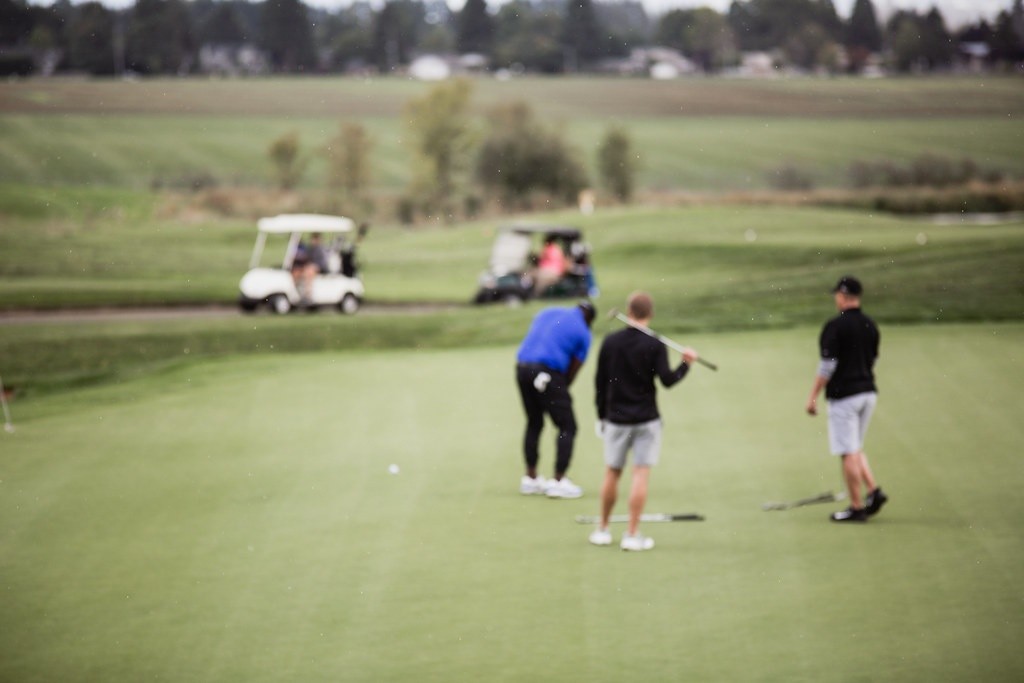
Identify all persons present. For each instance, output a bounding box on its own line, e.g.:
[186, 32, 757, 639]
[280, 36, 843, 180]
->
[590, 293, 694, 552]
[512, 300, 595, 497]
[807, 275, 889, 522]
[293, 232, 345, 290]
[529, 236, 568, 292]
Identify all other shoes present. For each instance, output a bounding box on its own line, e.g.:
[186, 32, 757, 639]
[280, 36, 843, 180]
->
[832, 506, 867, 520]
[621, 534, 655, 551]
[590, 531, 612, 545]
[864, 487, 887, 513]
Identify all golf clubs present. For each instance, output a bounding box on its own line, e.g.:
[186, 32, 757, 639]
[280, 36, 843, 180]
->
[577, 513, 704, 524]
[606, 307, 719, 374]
[763, 492, 835, 513]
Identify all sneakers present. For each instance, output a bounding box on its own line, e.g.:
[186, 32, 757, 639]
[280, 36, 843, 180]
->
[546, 477, 582, 498]
[521, 475, 547, 494]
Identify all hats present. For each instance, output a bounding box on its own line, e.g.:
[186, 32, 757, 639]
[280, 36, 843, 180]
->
[830, 275, 862, 295]
[579, 301, 595, 327]
